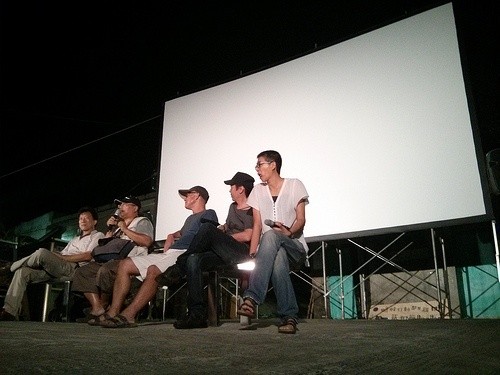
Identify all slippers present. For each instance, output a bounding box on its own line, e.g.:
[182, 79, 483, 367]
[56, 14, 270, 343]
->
[88, 311, 111, 325]
[102, 313, 137, 328]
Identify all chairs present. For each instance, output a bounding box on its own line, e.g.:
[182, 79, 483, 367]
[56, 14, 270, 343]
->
[43, 239, 87, 322]
[147, 239, 251, 329]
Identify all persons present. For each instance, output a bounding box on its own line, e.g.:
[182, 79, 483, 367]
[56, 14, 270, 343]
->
[155, 171, 255, 329]
[236, 150, 310, 334]
[71, 195, 153, 323]
[88, 186, 218, 328]
[0, 207, 105, 320]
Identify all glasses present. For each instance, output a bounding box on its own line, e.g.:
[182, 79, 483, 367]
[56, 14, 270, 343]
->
[255, 161, 273, 168]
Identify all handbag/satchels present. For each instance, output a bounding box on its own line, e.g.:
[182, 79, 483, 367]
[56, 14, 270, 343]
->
[90, 236, 134, 261]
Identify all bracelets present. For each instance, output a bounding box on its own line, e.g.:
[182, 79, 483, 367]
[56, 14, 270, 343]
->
[289, 231, 292, 236]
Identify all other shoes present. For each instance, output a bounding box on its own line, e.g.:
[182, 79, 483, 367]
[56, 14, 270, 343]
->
[75, 312, 95, 323]
[174, 313, 207, 329]
[0, 312, 12, 321]
[177, 251, 191, 263]
[0, 266, 9, 274]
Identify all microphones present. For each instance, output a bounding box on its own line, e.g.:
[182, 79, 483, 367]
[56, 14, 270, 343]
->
[264, 219, 290, 230]
[107, 209, 120, 230]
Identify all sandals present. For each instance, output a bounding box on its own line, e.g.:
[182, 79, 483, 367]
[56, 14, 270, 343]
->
[278, 319, 296, 334]
[238, 303, 255, 317]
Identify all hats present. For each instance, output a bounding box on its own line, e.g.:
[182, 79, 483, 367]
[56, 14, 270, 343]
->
[179, 185, 208, 202]
[224, 172, 254, 188]
[113, 196, 141, 209]
